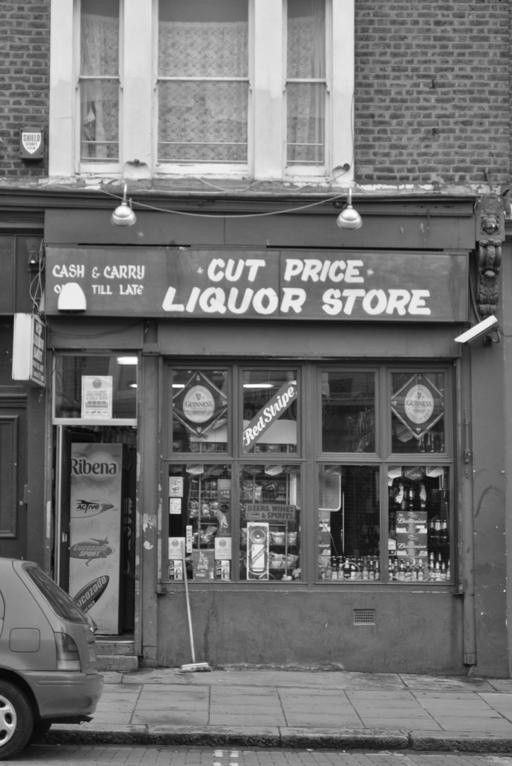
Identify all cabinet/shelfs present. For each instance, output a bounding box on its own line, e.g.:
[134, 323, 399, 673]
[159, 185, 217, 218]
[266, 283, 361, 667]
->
[186, 442, 299, 581]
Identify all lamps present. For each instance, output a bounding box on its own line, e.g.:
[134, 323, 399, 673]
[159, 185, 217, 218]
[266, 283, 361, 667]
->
[334, 188, 364, 232]
[111, 184, 137, 228]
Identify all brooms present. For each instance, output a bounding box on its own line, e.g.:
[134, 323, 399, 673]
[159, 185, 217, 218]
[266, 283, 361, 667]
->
[180, 541, 212, 672]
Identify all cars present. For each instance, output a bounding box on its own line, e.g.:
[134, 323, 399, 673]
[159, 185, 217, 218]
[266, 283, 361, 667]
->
[0, 553, 103, 760]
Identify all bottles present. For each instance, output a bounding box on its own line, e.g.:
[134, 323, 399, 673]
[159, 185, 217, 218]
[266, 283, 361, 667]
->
[397, 483, 428, 511]
[329, 551, 451, 582]
[428, 518, 449, 540]
[418, 431, 445, 452]
